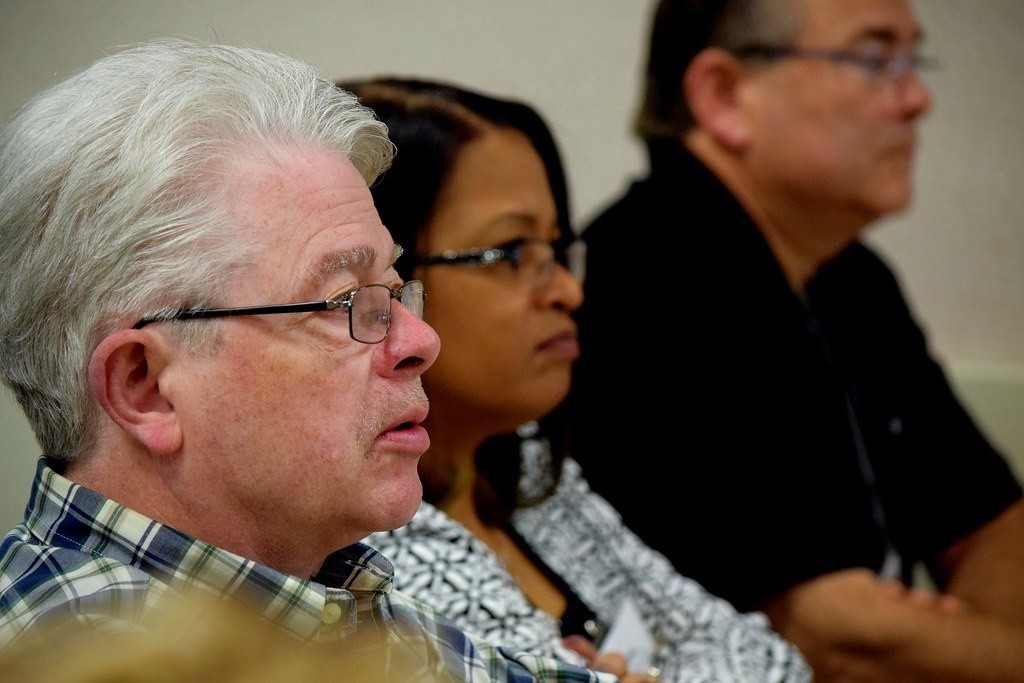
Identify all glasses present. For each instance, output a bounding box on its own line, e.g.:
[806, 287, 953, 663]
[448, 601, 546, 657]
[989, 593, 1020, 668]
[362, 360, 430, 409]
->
[132, 279, 426, 344]
[736, 43, 941, 97]
[405, 236, 589, 284]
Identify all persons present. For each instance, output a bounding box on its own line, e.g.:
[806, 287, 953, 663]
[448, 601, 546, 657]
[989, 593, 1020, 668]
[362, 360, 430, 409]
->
[534, 2, 1022, 683]
[333, 76, 815, 683]
[0, 39, 600, 683]
